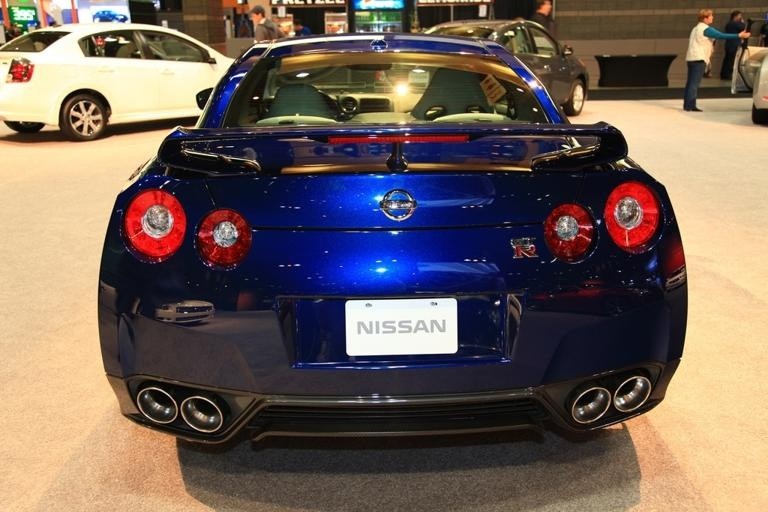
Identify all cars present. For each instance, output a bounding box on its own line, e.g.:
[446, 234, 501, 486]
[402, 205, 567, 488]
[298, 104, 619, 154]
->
[407, 17, 590, 117]
[0, 21, 237, 143]
[730, 16, 767, 127]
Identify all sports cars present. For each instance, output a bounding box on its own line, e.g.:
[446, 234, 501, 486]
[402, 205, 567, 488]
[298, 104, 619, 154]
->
[95, 33, 689, 447]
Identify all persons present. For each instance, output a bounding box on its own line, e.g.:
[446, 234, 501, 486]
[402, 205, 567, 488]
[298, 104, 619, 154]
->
[720, 10, 745, 79]
[294, 19, 312, 36]
[249, 5, 286, 41]
[760, 23, 768, 47]
[682, 8, 751, 112]
[528, 0, 552, 29]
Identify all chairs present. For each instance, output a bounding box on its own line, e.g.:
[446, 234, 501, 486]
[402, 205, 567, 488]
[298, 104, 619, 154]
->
[256, 67, 519, 124]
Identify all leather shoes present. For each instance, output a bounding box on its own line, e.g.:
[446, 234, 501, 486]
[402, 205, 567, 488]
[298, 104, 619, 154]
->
[684, 106, 703, 112]
